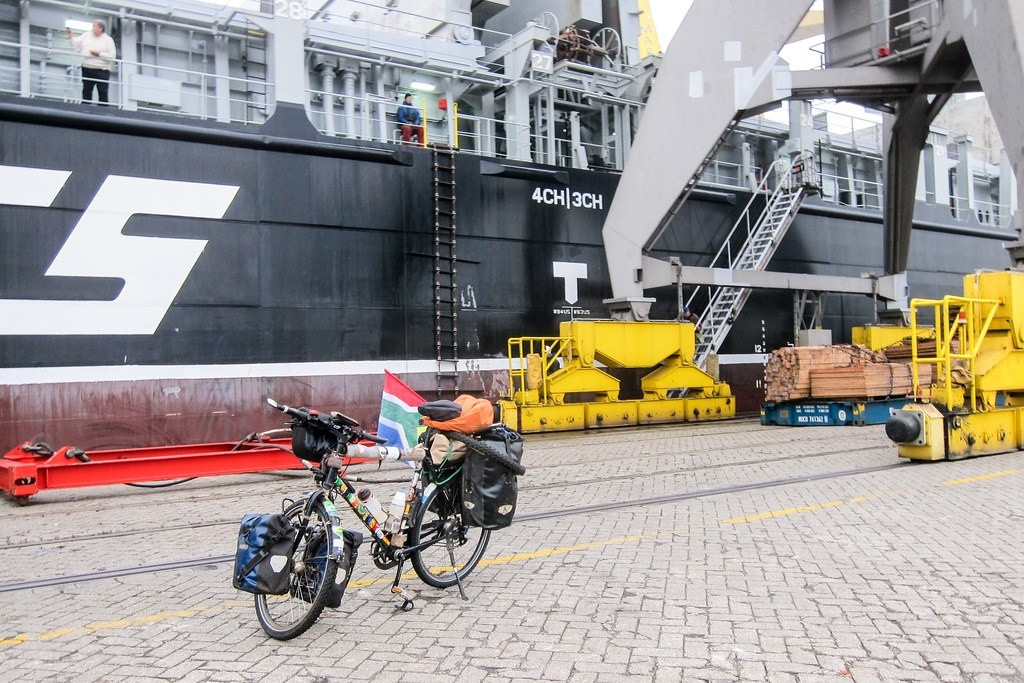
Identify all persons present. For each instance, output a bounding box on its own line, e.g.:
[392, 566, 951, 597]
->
[397, 90, 425, 145]
[63, 20, 117, 106]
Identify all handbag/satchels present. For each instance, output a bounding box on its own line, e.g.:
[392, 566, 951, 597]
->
[292, 529, 363, 608]
[460, 436, 525, 530]
[230, 511, 291, 595]
[290, 407, 347, 463]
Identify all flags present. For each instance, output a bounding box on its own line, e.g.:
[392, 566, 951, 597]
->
[373, 370, 433, 470]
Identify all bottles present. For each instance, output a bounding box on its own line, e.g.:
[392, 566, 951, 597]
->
[358, 488, 388, 524]
[385, 491, 406, 533]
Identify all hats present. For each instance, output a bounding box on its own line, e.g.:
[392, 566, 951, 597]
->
[405, 93, 412, 100]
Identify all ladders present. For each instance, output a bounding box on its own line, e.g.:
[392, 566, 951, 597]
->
[431, 145, 460, 399]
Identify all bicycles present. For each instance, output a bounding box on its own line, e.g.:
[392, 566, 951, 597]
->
[254, 400, 525, 643]
